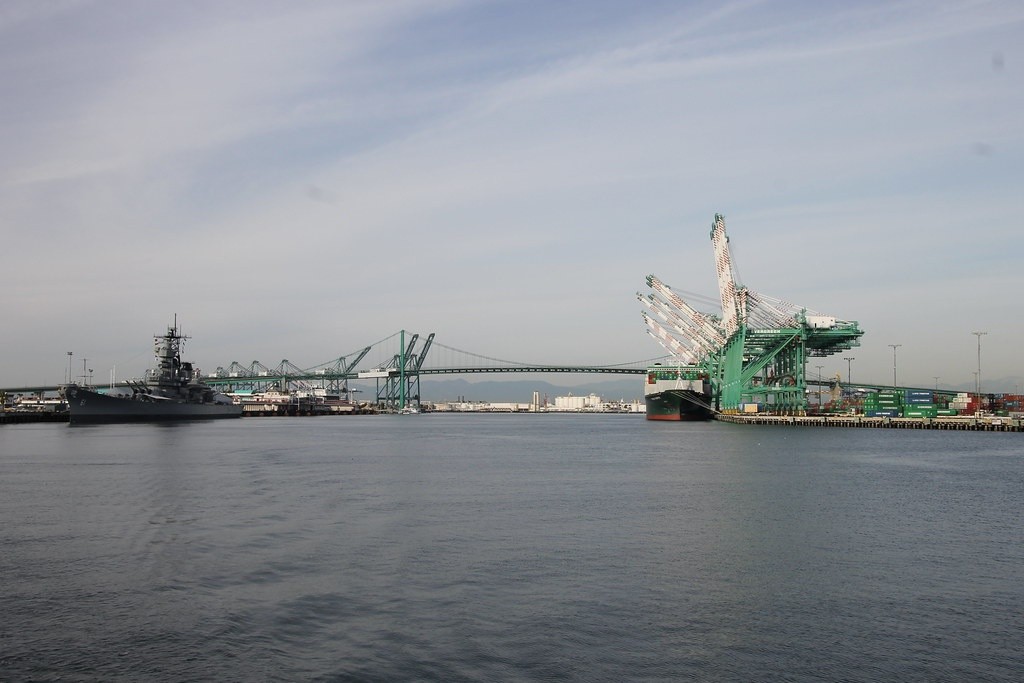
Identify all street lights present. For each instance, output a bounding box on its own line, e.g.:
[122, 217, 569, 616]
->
[972, 332, 987, 412]
[815, 365, 824, 405]
[933, 377, 940, 395]
[844, 357, 855, 404]
[888, 344, 902, 393]
[972, 371, 978, 397]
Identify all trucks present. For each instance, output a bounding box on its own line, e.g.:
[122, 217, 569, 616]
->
[805, 316, 839, 330]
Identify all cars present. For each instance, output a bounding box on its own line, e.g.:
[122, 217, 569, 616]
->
[835, 319, 854, 330]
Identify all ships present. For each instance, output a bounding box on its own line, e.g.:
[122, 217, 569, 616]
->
[58, 313, 245, 426]
[645, 361, 712, 421]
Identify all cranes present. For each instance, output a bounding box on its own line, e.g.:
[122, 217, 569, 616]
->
[636, 212, 866, 418]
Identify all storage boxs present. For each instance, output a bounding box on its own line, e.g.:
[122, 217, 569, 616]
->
[863, 390, 1024, 418]
[806, 399, 863, 415]
[648, 371, 710, 384]
